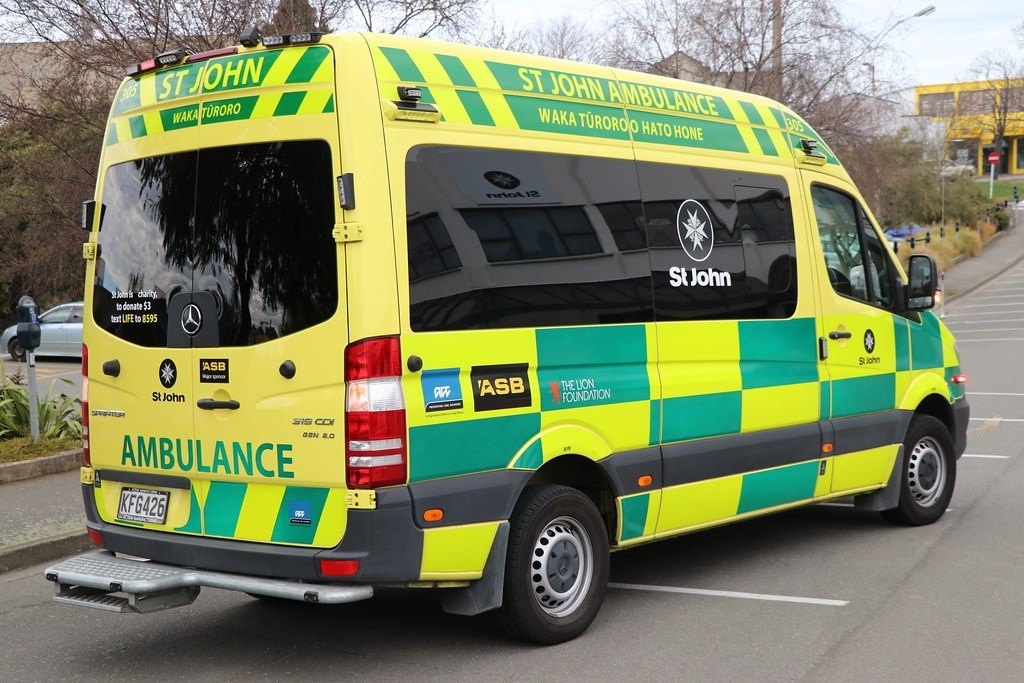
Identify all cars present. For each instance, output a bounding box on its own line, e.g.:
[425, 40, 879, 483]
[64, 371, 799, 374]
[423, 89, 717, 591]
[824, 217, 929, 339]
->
[0, 302, 84, 363]
[883, 221, 924, 237]
[820, 238, 871, 267]
[924, 159, 975, 181]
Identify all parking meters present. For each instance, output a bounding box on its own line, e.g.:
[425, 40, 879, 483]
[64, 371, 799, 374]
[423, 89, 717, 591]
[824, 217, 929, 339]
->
[15, 296, 41, 439]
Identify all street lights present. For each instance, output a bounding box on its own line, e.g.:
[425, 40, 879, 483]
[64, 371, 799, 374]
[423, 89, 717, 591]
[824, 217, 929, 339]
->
[871, 6, 935, 223]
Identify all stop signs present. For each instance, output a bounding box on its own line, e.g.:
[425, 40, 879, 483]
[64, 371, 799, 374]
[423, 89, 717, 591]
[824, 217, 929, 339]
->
[988, 152, 999, 164]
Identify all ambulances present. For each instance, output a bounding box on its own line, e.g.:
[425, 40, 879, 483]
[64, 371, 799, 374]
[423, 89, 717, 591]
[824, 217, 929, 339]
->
[44, 27, 970, 645]
